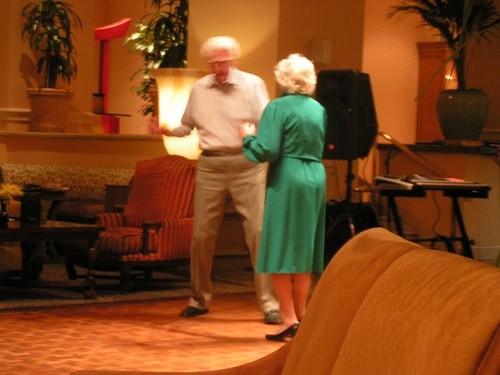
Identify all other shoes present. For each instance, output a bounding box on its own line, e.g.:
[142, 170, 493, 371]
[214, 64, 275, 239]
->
[180, 306, 208, 318]
[264, 311, 284, 324]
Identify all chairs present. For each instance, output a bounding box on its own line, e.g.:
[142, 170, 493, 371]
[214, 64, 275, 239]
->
[214, 228, 500, 375]
[87, 156, 198, 285]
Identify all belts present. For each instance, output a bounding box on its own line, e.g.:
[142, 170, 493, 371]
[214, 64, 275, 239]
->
[201, 150, 242, 157]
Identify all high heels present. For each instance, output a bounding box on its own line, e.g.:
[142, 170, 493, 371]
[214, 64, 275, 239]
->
[266, 323, 299, 340]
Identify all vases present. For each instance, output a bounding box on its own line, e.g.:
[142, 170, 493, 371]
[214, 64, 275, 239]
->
[0, 198, 9, 222]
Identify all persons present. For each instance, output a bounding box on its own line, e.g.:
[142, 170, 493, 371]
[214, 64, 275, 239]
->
[151, 36, 287, 324]
[239, 54, 328, 341]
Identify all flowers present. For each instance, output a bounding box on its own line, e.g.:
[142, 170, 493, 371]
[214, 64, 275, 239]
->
[0, 182, 25, 201]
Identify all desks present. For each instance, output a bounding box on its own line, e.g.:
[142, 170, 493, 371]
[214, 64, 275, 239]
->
[351, 182, 491, 258]
[0, 220, 105, 298]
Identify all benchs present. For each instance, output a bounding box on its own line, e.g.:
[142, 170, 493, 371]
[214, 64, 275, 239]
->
[0, 162, 135, 253]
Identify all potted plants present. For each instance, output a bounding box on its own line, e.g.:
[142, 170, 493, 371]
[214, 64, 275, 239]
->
[25, 0, 82, 131]
[385, 0, 500, 141]
